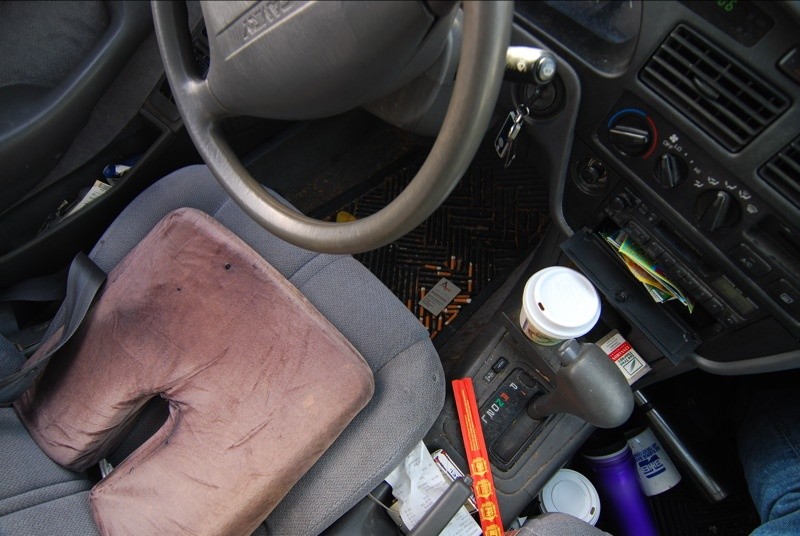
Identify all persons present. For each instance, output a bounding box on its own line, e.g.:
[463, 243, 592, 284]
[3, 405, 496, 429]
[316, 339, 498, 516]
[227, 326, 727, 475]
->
[734, 388, 800, 536]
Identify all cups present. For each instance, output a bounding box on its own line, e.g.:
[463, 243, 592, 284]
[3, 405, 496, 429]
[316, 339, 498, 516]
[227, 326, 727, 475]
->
[628, 427, 682, 496]
[537, 469, 601, 526]
[583, 442, 659, 536]
[520, 265, 601, 346]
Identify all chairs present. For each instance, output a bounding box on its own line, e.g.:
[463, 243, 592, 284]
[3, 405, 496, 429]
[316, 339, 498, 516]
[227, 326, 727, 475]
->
[0, 164, 446, 536]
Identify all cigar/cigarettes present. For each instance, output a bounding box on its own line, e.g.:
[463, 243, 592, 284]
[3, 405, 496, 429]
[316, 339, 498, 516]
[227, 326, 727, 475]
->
[407, 255, 472, 339]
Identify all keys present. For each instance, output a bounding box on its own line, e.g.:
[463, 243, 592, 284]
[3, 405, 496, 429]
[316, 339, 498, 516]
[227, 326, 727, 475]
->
[521, 85, 550, 112]
